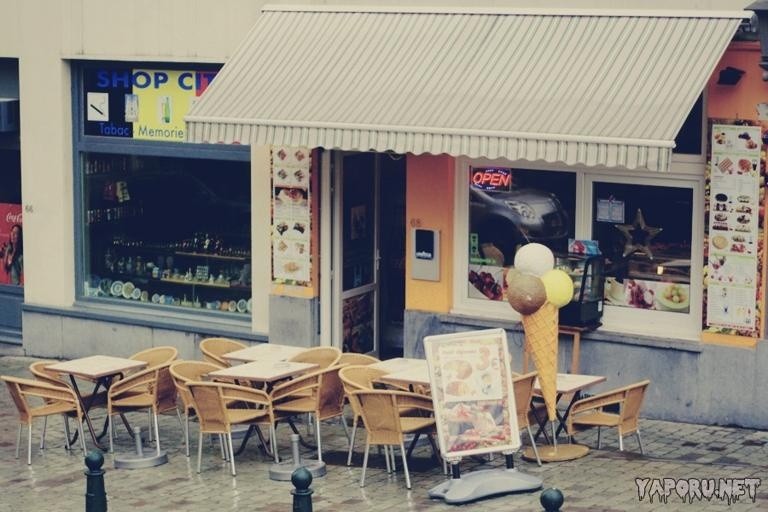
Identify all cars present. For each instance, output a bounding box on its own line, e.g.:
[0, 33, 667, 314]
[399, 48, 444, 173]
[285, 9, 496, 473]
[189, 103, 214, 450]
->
[470, 184, 571, 240]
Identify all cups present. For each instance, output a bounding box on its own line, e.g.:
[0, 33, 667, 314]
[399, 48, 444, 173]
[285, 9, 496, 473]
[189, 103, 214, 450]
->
[611, 278, 629, 302]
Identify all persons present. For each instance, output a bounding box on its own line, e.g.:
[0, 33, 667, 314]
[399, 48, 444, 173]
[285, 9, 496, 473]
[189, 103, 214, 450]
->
[0, 223, 23, 285]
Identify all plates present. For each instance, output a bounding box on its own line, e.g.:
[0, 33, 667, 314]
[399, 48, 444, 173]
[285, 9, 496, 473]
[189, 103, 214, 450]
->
[656, 284, 689, 309]
[606, 285, 635, 307]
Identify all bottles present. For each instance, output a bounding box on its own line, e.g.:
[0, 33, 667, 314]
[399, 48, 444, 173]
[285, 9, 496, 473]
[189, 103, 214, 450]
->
[85, 157, 145, 224]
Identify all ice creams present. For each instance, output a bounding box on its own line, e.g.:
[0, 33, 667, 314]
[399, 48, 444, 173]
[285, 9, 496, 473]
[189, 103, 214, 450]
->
[507, 243, 574, 423]
